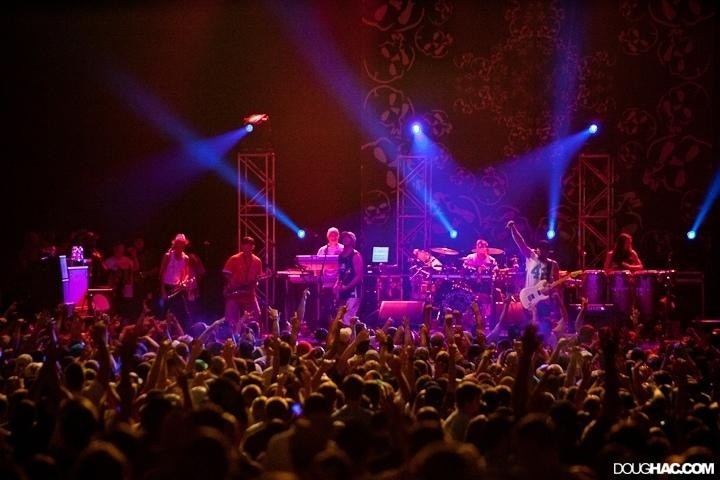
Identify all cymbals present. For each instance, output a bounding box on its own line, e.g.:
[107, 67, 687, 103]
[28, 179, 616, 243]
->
[431, 247, 458, 256]
[472, 248, 504, 255]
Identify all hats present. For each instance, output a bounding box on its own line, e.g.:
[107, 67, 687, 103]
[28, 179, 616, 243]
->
[327, 227, 339, 239]
[171, 233, 189, 246]
[340, 231, 356, 242]
[534, 239, 554, 254]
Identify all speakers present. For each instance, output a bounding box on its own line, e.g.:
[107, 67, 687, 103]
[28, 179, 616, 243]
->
[568, 302, 630, 335]
[379, 300, 426, 328]
[38, 255, 91, 318]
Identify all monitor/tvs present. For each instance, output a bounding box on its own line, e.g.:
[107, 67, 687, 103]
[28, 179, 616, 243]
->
[372, 246, 390, 264]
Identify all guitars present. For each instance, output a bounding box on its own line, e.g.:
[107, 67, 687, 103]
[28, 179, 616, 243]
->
[519, 270, 583, 310]
[154, 278, 196, 311]
[224, 268, 272, 295]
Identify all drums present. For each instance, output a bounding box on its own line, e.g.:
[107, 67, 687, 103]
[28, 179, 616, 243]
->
[442, 284, 475, 314]
[582, 269, 608, 304]
[606, 271, 634, 314]
[656, 270, 675, 299]
[632, 269, 658, 316]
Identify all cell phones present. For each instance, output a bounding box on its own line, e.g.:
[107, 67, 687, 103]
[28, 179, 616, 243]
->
[291, 402, 303, 416]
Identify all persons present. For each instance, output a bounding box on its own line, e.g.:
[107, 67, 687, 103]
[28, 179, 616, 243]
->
[1, 216, 720, 480]
